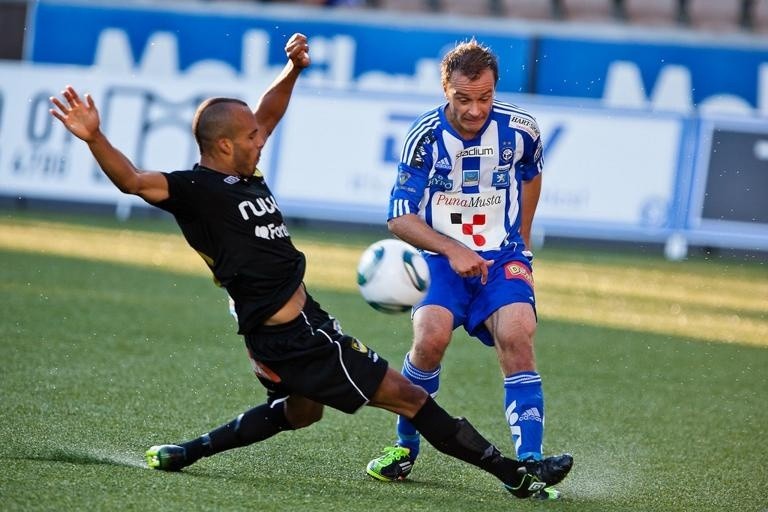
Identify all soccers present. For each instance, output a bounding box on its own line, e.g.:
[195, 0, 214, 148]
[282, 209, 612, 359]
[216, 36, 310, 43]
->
[356, 239, 430, 313]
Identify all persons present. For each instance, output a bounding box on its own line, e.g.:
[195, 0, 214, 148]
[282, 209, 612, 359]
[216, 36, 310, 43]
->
[46, 28, 576, 503]
[365, 36, 571, 502]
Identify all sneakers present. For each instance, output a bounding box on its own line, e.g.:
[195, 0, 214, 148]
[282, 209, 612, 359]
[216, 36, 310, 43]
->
[503, 453, 574, 501]
[145, 444, 185, 470]
[366, 446, 414, 482]
